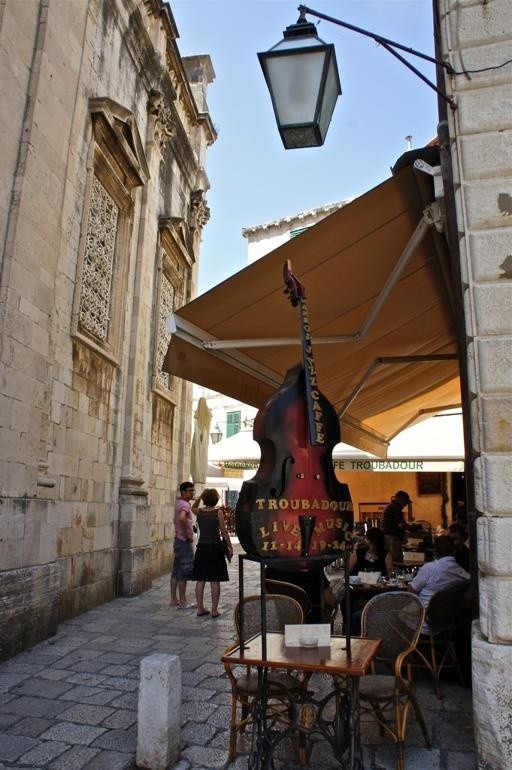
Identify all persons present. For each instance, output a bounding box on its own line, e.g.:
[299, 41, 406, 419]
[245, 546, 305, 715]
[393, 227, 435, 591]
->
[168, 482, 197, 609]
[448, 524, 469, 574]
[191, 488, 233, 617]
[388, 535, 472, 675]
[349, 528, 393, 636]
[383, 490, 413, 561]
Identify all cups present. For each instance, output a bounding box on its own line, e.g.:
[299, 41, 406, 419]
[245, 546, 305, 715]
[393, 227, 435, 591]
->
[349, 568, 413, 588]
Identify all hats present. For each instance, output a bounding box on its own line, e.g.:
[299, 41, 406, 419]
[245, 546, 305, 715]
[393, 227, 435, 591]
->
[395, 490, 412, 504]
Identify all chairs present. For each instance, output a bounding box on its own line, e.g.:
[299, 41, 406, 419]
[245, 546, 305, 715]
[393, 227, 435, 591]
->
[416, 520, 431, 531]
[265, 579, 313, 624]
[221, 594, 312, 769]
[406, 579, 467, 699]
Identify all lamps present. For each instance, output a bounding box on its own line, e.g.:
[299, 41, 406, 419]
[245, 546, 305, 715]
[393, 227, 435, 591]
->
[258, 2, 457, 151]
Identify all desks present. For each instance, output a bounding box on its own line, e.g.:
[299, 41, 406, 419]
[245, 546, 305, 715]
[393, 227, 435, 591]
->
[221, 630, 383, 770]
[335, 591, 432, 770]
[343, 579, 411, 593]
[392, 552, 425, 572]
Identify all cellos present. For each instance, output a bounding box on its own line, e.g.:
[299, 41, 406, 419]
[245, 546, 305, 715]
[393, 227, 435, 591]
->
[235, 260, 354, 573]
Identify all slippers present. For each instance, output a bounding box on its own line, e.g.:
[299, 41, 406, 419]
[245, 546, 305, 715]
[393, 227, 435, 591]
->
[212, 610, 221, 618]
[168, 601, 180, 606]
[177, 602, 197, 609]
[197, 609, 210, 616]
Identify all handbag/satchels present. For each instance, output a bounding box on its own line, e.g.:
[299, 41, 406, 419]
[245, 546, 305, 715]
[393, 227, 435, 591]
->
[221, 537, 232, 563]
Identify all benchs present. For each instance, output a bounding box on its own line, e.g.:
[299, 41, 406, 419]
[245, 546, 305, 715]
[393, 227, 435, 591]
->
[402, 536, 424, 551]
